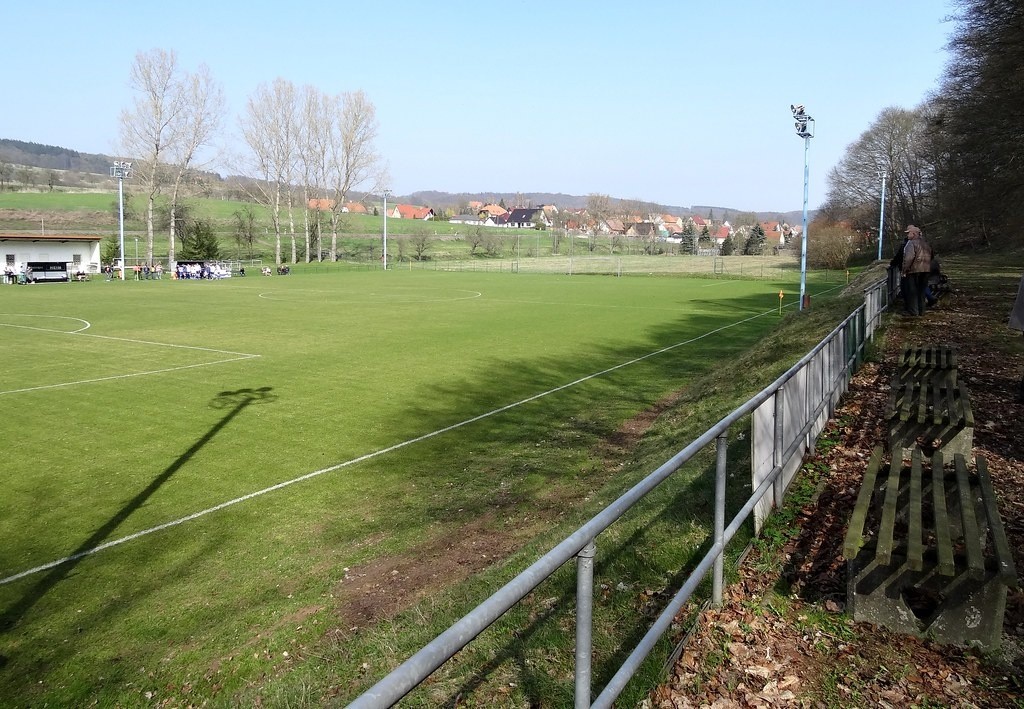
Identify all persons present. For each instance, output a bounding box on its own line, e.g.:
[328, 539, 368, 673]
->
[176, 263, 221, 280]
[277, 265, 290, 275]
[264, 267, 272, 276]
[4, 263, 33, 285]
[77, 271, 85, 281]
[890, 225, 941, 317]
[133, 261, 163, 280]
[105, 264, 113, 281]
[240, 268, 245, 277]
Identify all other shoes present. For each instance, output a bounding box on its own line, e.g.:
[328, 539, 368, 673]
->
[902, 311, 919, 317]
[925, 298, 938, 306]
[918, 309, 925, 316]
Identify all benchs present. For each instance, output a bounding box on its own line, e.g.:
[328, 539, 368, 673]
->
[886, 373, 975, 467]
[899, 345, 958, 389]
[843, 446, 1016, 651]
[73, 273, 90, 282]
[930, 275, 951, 305]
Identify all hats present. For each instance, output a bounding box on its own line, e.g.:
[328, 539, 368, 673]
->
[904, 227, 921, 233]
